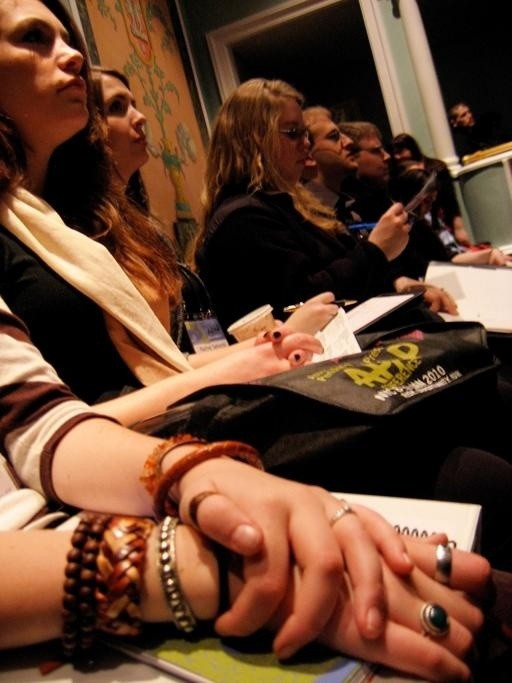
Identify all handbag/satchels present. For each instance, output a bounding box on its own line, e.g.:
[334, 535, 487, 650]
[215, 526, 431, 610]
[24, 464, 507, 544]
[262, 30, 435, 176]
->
[159, 321, 501, 481]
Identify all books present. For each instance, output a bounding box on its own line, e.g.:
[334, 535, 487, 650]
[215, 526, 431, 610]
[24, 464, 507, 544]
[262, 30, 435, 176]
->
[116, 626, 368, 683]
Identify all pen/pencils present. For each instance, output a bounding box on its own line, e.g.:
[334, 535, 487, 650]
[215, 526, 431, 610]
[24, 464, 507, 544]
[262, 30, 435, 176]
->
[283, 299, 358, 313]
[348, 218, 415, 231]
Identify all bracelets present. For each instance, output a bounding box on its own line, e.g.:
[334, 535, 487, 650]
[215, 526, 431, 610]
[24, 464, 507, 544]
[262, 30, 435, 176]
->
[59, 432, 264, 668]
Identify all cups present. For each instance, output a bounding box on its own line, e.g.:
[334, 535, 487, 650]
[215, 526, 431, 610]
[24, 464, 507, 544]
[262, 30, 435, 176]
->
[227, 303, 277, 344]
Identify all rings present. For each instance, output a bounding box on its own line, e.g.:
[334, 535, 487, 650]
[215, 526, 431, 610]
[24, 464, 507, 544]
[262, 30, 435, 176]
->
[434, 540, 452, 583]
[328, 504, 354, 525]
[419, 600, 451, 640]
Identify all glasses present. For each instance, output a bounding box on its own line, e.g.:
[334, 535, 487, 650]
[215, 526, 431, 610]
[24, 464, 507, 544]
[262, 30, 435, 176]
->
[278, 126, 299, 139]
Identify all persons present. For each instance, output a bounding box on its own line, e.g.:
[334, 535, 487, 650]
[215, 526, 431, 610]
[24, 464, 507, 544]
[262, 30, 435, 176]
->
[0, 303, 493, 683]
[300, 101, 512, 279]
[196, 79, 459, 346]
[0, 4, 328, 426]
[52, 63, 339, 371]
